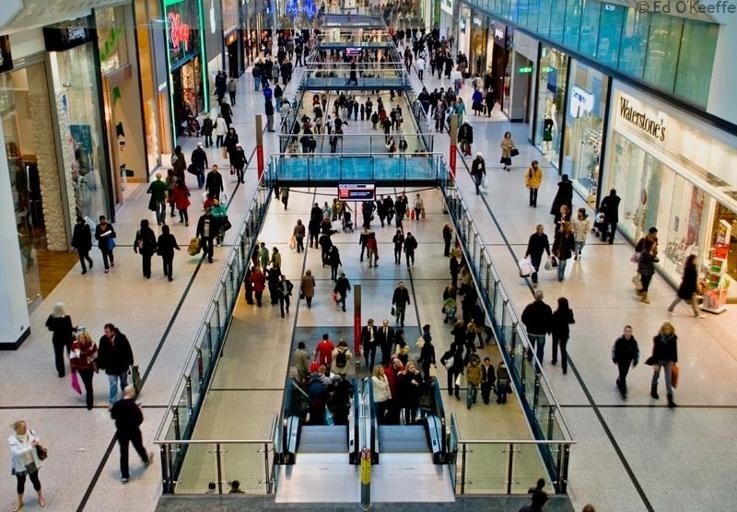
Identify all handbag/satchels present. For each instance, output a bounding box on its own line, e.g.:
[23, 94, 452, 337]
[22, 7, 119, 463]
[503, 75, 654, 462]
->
[188, 238, 201, 256]
[36, 444, 48, 460]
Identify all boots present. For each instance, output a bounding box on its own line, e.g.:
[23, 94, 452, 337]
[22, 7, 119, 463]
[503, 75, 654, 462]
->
[667, 393, 678, 409]
[651, 384, 659, 399]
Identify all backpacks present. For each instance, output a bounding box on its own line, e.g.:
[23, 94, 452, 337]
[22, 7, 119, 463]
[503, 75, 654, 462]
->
[336, 348, 347, 368]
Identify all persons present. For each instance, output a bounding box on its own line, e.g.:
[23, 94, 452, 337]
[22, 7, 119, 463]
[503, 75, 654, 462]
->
[645, 322, 678, 408]
[6, 420, 45, 512]
[667, 254, 708, 318]
[44, 0, 660, 511]
